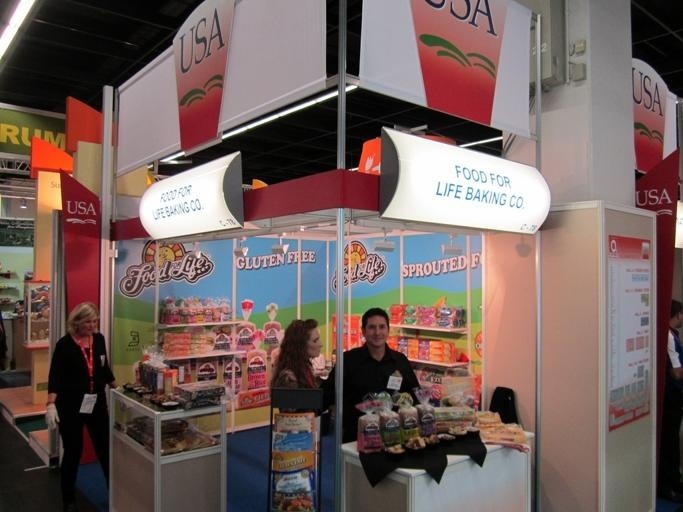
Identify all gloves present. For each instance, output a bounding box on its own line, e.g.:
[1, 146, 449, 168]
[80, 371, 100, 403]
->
[45, 404, 60, 430]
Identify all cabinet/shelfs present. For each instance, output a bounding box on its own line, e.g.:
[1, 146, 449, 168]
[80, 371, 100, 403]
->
[389, 322, 469, 368]
[157, 320, 247, 362]
[0, 272, 16, 305]
[22, 280, 52, 349]
[109, 388, 227, 512]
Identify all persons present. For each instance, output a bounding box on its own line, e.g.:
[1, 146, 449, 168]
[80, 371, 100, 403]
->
[45, 302, 118, 512]
[271, 319, 330, 418]
[15, 300, 25, 313]
[295, 308, 421, 443]
[658, 299, 683, 504]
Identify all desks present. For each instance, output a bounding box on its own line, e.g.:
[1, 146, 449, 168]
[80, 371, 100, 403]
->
[339, 431, 535, 512]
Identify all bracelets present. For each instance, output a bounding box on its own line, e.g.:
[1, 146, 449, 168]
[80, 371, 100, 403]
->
[47, 401, 56, 406]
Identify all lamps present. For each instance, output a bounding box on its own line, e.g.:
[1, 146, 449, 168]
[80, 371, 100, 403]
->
[189, 232, 463, 259]
